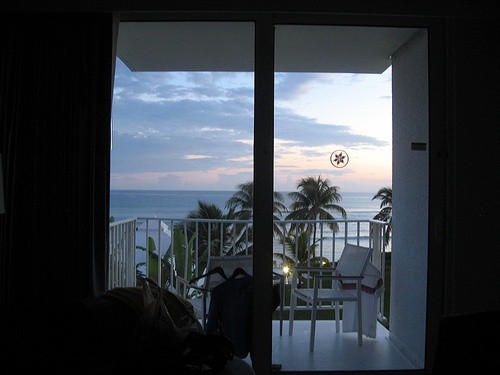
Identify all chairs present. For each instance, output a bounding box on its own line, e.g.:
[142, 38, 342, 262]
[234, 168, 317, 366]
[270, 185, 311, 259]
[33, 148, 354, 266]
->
[287, 244, 375, 352]
[173, 253, 257, 328]
